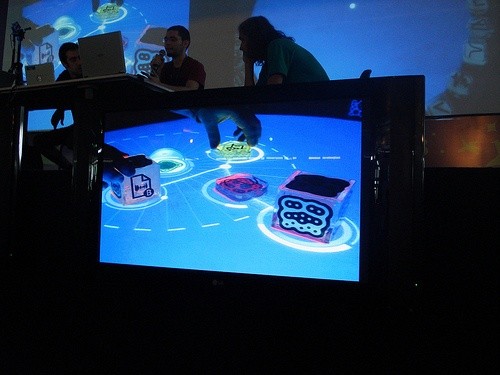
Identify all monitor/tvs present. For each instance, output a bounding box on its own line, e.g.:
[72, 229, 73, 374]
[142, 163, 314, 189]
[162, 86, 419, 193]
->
[25, 63, 56, 85]
[92, 74, 424, 295]
[78, 31, 126, 78]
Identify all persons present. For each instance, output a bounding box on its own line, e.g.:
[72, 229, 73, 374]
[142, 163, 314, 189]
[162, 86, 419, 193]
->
[239, 16, 329, 86]
[51, 42, 100, 134]
[142, 25, 206, 91]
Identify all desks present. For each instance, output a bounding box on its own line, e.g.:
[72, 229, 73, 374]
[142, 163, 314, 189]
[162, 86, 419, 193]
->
[0, 73, 175, 242]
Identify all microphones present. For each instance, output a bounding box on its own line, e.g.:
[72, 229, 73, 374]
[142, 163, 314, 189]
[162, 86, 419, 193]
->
[154, 50, 165, 70]
